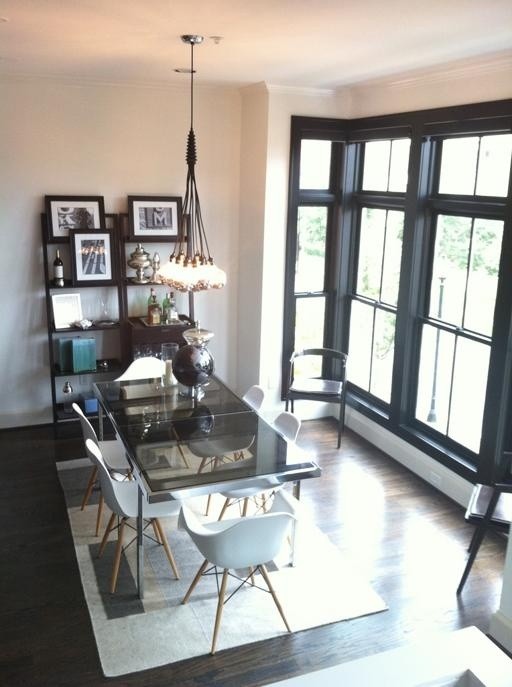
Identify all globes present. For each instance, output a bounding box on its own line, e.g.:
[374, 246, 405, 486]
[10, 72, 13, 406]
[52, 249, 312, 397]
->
[171, 399, 215, 442]
[172, 344, 213, 398]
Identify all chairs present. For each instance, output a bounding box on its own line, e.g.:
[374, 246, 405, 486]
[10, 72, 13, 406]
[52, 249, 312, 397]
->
[188, 384, 262, 478]
[72, 401, 159, 536]
[287, 347, 350, 447]
[176, 489, 299, 656]
[112, 355, 188, 469]
[211, 410, 300, 520]
[75, 439, 182, 596]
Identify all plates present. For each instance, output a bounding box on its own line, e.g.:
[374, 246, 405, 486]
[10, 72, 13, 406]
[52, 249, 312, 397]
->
[93, 319, 115, 328]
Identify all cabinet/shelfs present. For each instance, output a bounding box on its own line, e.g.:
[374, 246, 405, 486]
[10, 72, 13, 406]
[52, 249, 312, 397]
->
[42, 215, 129, 437]
[114, 213, 194, 365]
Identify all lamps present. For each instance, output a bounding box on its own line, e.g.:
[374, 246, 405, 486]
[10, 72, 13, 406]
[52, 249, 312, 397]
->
[156, 34, 227, 293]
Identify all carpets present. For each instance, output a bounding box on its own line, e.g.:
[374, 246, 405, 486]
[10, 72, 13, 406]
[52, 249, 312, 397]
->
[53, 453, 388, 679]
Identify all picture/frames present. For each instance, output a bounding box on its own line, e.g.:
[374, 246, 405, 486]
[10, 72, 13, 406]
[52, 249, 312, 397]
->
[44, 195, 105, 243]
[67, 227, 118, 283]
[127, 194, 183, 239]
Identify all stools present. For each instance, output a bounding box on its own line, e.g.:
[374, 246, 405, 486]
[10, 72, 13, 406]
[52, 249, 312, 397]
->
[452, 451, 511, 594]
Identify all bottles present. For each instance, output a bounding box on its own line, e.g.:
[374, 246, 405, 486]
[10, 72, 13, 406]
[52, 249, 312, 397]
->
[147, 288, 179, 325]
[53, 249, 65, 287]
[64, 381, 74, 414]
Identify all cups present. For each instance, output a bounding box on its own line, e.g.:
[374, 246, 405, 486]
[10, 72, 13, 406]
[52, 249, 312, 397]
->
[134, 344, 160, 359]
[160, 387, 179, 425]
[161, 342, 179, 387]
